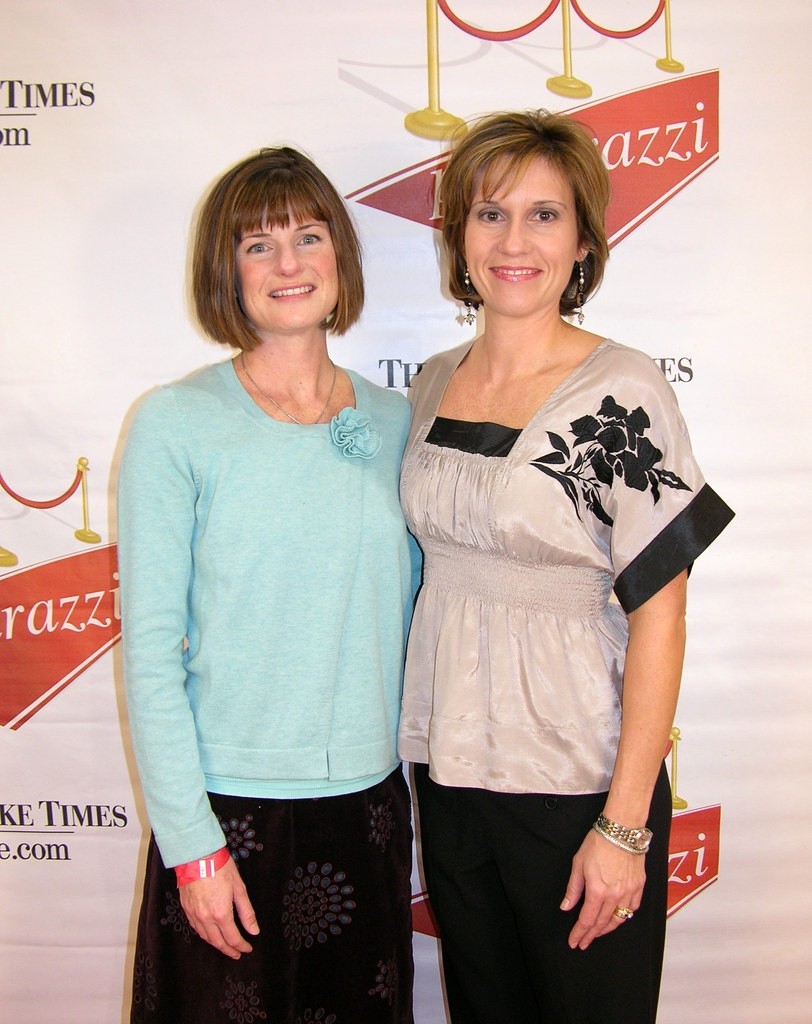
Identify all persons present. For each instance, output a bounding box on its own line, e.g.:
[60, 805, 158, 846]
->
[400, 109, 734, 1023]
[117, 147, 424, 1024]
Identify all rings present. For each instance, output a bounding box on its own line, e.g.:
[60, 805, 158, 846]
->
[615, 908, 633, 919]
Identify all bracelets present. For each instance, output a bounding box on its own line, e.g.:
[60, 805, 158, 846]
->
[173, 847, 231, 888]
[593, 823, 649, 854]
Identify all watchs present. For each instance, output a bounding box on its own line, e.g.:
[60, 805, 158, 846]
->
[597, 812, 653, 850]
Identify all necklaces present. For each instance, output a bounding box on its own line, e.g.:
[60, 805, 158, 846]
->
[241, 350, 336, 425]
